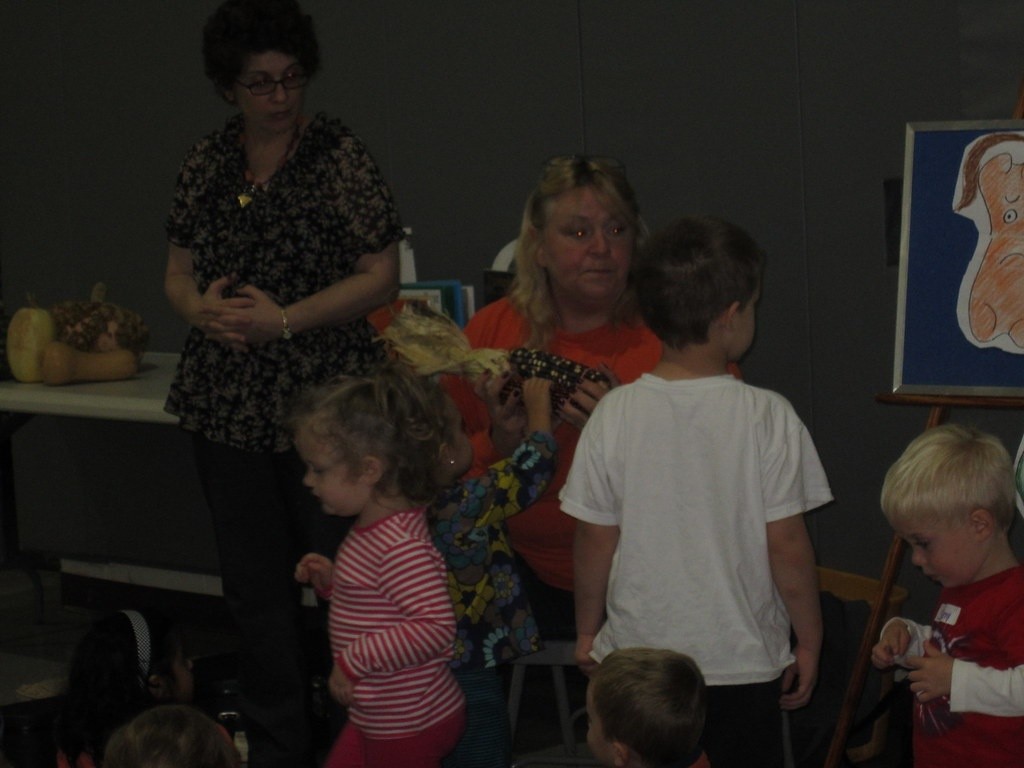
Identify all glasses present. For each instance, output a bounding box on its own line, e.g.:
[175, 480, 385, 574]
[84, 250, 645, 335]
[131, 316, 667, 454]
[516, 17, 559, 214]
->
[231, 62, 312, 96]
[540, 154, 627, 189]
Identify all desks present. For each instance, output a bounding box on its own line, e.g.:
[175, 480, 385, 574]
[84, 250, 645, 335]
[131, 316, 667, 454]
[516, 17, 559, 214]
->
[0, 351, 179, 566]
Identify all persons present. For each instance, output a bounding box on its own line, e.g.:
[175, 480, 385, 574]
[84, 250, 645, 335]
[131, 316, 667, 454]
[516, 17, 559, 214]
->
[587, 648, 710, 768]
[438, 156, 742, 768]
[559, 219, 834, 768]
[293, 361, 467, 768]
[416, 379, 558, 768]
[872, 425, 1024, 768]
[104, 707, 240, 768]
[164, 0, 404, 768]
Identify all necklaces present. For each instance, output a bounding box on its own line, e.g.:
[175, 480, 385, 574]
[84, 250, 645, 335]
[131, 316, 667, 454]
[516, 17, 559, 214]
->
[240, 124, 300, 186]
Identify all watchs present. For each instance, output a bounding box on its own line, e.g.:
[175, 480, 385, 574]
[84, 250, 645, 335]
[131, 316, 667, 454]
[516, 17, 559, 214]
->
[280, 308, 292, 342]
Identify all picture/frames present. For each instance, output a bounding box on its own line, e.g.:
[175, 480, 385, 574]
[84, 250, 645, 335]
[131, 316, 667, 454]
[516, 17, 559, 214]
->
[890, 118, 1024, 397]
